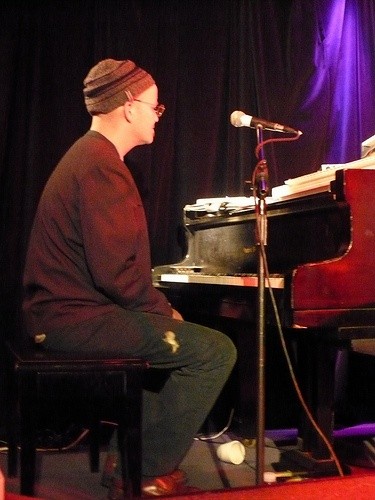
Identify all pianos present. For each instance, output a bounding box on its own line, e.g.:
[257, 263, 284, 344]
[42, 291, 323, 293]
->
[152, 168, 375, 463]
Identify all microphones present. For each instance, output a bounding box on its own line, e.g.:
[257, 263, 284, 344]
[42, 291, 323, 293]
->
[230, 110, 298, 135]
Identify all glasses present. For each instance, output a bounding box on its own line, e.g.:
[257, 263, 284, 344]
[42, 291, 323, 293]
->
[133, 98, 165, 117]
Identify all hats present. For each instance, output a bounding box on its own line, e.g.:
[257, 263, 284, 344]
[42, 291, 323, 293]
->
[83, 59, 155, 113]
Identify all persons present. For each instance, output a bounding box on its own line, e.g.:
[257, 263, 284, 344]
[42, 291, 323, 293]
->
[22, 57, 238, 497]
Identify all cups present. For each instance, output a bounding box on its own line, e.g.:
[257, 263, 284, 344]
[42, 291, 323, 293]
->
[216, 440, 245, 465]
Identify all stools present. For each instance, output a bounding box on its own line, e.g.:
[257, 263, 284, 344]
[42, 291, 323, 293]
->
[6, 341, 151, 500]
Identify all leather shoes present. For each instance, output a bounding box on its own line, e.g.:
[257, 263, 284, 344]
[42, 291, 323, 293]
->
[107, 477, 202, 499]
[100, 454, 188, 488]
